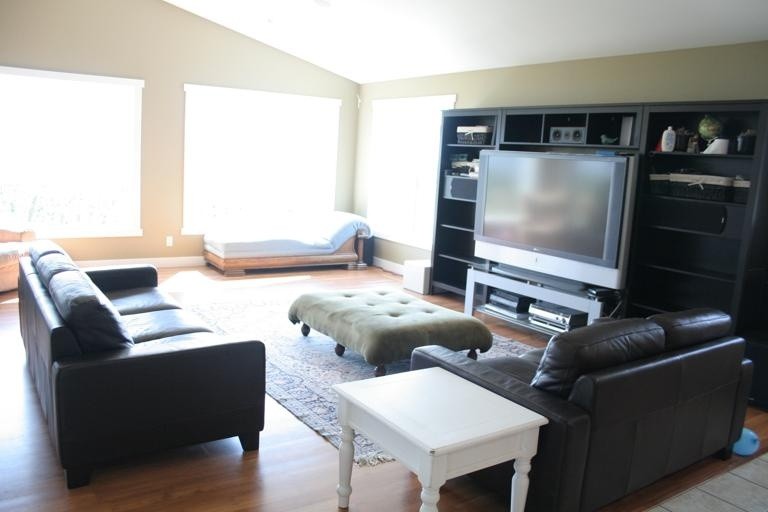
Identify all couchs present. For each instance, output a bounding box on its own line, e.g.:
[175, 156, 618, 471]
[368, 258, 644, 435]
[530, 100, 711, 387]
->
[0, 229, 33, 292]
[285, 284, 493, 378]
[410, 309, 754, 509]
[10, 242, 266, 491]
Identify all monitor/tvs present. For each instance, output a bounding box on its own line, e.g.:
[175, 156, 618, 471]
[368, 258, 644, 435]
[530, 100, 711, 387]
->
[473, 149, 638, 293]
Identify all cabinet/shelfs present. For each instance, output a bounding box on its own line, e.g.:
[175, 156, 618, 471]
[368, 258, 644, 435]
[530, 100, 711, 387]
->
[499, 103, 643, 154]
[462, 268, 626, 339]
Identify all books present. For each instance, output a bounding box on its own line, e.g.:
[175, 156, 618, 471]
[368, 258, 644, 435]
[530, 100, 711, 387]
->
[482, 289, 529, 320]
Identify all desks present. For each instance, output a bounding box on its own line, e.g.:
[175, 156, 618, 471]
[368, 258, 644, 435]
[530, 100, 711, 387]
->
[330, 368, 549, 510]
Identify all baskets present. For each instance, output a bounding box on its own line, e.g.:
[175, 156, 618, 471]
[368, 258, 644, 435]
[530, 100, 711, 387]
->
[456, 126, 493, 145]
[732, 179, 751, 204]
[668, 173, 734, 202]
[649, 174, 670, 195]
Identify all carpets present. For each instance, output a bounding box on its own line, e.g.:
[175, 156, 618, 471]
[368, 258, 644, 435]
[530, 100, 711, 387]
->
[188, 300, 537, 468]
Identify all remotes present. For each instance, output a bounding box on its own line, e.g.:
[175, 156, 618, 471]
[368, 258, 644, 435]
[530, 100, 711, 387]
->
[586, 287, 615, 295]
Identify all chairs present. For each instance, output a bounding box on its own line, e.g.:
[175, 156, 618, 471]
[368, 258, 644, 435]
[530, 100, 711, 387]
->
[623, 98, 766, 411]
[428, 106, 500, 300]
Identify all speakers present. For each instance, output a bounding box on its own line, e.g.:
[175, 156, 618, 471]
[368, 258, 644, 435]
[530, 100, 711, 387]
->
[549, 126, 586, 143]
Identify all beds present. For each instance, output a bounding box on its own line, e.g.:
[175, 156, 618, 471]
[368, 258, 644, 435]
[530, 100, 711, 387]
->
[201, 213, 372, 278]
